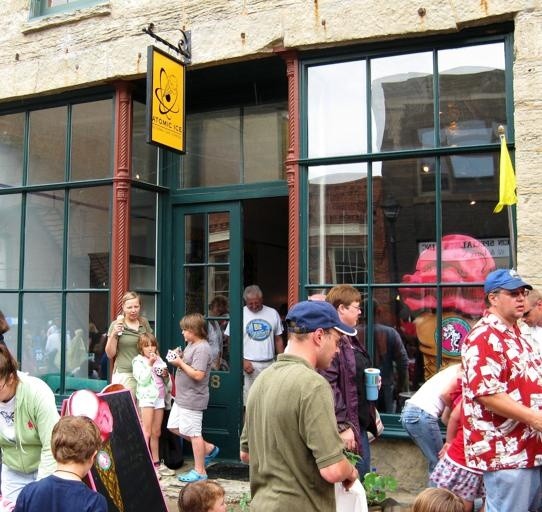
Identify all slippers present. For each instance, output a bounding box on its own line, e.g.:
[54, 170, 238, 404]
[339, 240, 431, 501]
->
[178, 469, 210, 483]
[203, 444, 220, 467]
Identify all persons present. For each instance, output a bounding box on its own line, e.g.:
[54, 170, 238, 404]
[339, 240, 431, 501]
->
[351, 294, 416, 423]
[166, 312, 219, 482]
[238, 303, 358, 511]
[460, 268, 541, 512]
[401, 363, 466, 491]
[177, 480, 227, 512]
[45, 318, 70, 375]
[1, 342, 61, 512]
[519, 285, 540, 356]
[313, 288, 381, 488]
[12, 416, 108, 512]
[132, 332, 177, 480]
[206, 296, 227, 371]
[412, 487, 465, 512]
[225, 285, 285, 424]
[428, 396, 488, 512]
[105, 292, 152, 426]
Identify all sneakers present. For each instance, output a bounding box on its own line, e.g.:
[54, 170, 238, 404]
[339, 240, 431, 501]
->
[155, 461, 176, 476]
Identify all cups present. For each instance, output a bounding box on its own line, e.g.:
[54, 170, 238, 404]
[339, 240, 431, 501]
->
[156, 366, 165, 375]
[363, 366, 381, 401]
[165, 349, 177, 361]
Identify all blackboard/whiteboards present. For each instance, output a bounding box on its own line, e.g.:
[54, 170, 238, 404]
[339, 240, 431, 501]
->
[66, 388, 169, 512]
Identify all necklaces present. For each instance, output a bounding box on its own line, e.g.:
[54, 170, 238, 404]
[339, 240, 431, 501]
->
[53, 470, 82, 480]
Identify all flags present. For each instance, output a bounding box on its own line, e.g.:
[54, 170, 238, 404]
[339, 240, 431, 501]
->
[493, 132, 519, 215]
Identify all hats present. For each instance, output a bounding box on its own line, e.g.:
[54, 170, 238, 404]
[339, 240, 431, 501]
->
[283, 297, 362, 338]
[482, 268, 536, 293]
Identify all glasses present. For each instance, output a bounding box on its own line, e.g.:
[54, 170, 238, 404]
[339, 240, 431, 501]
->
[494, 290, 531, 298]
